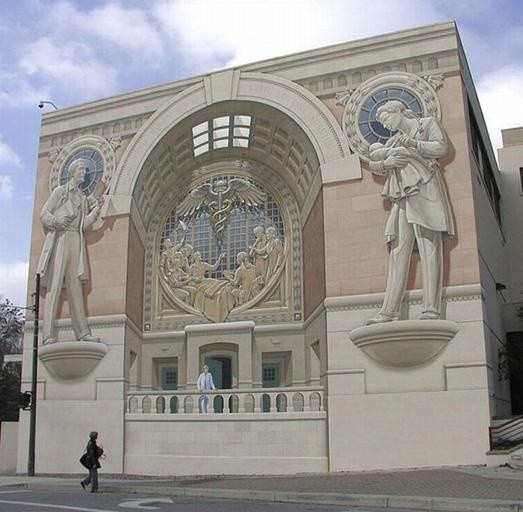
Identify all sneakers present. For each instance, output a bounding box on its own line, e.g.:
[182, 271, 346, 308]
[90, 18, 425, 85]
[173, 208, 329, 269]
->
[80, 481, 85, 489]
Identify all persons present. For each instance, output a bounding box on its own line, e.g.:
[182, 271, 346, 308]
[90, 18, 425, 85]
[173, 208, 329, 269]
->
[160, 225, 285, 312]
[79, 431, 103, 492]
[34, 157, 107, 345]
[367, 99, 455, 324]
[197, 364, 216, 413]
